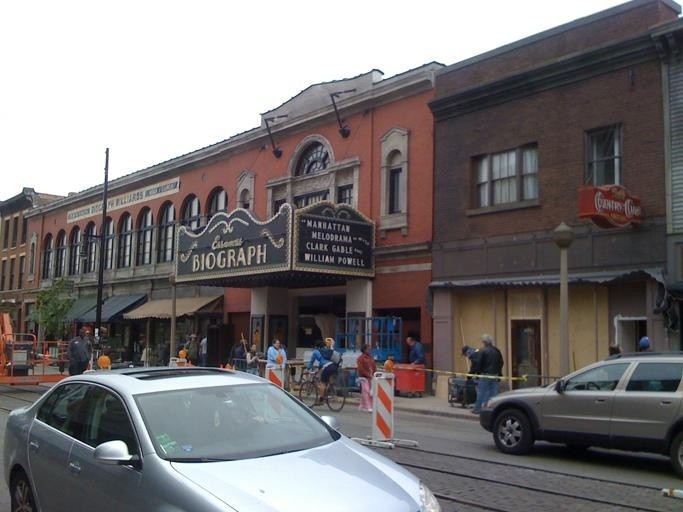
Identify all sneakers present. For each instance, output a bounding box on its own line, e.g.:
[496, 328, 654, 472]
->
[314, 400, 323, 405]
[368, 409, 372, 413]
[358, 407, 367, 411]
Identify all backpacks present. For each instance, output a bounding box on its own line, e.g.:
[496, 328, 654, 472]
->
[320, 348, 342, 364]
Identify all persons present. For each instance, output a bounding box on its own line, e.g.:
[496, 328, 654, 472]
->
[461, 345, 481, 382]
[245, 343, 267, 376]
[403, 337, 425, 365]
[265, 339, 286, 389]
[234, 339, 249, 359]
[476, 335, 504, 412]
[354, 344, 376, 412]
[69, 326, 92, 375]
[185, 334, 202, 367]
[306, 340, 339, 405]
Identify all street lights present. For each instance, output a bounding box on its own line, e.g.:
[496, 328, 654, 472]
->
[80, 148, 110, 350]
[168, 273, 180, 357]
[555, 221, 577, 377]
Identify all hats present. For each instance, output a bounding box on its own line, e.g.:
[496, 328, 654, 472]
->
[461, 345, 468, 355]
[639, 336, 649, 349]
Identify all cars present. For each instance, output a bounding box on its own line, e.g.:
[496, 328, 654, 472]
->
[1, 368, 440, 512]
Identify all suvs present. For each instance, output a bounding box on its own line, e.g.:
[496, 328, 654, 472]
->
[479, 351, 683, 477]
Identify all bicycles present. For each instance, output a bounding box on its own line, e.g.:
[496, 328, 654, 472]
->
[299, 371, 346, 412]
